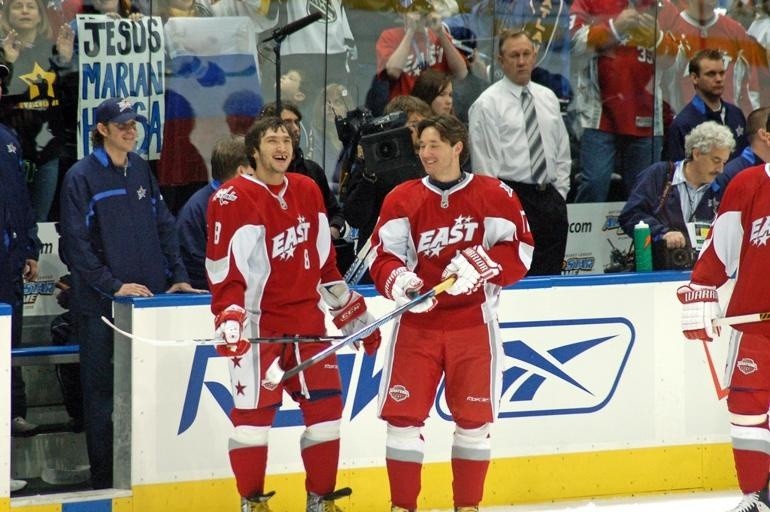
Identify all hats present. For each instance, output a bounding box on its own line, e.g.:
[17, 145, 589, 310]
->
[98, 97, 146, 125]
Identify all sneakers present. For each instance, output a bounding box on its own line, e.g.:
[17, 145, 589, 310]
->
[730, 489, 770, 512]
[305, 487, 350, 512]
[240, 492, 275, 511]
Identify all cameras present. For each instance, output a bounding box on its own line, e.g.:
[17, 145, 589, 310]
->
[663, 243, 699, 270]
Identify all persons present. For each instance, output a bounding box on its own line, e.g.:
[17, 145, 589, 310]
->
[1, 0, 770, 488]
[368, 116, 535, 511]
[205, 120, 381, 511]
[681, 113, 770, 511]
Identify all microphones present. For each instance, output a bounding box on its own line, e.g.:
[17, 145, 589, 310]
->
[261, 12, 323, 44]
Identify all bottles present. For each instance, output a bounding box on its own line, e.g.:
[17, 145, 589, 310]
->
[633, 219, 654, 274]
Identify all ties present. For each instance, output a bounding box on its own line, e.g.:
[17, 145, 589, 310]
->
[521, 86, 548, 186]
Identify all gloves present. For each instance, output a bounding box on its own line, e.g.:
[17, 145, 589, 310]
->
[215, 304, 250, 355]
[676, 284, 722, 341]
[329, 291, 381, 355]
[385, 267, 439, 314]
[442, 245, 501, 296]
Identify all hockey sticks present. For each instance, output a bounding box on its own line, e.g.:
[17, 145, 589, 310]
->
[266, 275, 455, 388]
[100, 314, 345, 344]
